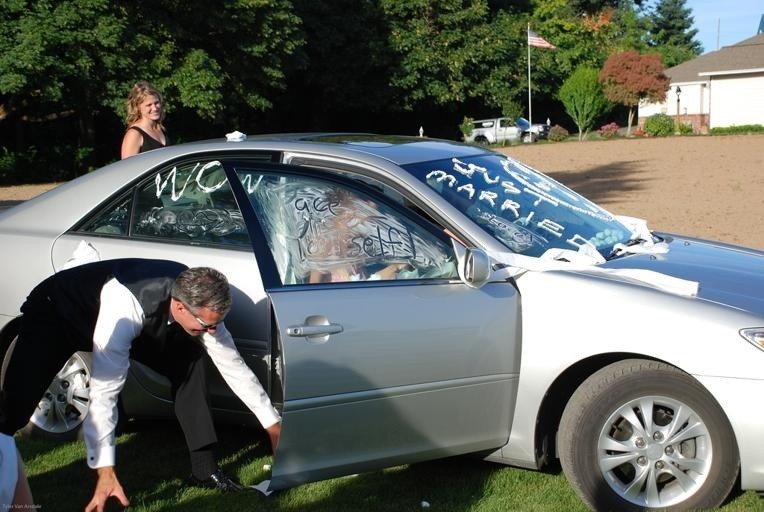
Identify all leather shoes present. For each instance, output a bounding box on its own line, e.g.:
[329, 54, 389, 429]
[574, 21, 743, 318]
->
[185, 469, 242, 494]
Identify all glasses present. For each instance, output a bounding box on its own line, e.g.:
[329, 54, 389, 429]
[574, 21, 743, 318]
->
[171, 295, 224, 328]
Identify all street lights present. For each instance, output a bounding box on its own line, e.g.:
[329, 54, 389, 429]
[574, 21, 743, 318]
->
[674, 85, 682, 136]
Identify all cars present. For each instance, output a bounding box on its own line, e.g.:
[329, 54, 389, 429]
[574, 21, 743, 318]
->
[0, 131, 764, 512]
[463, 116, 552, 147]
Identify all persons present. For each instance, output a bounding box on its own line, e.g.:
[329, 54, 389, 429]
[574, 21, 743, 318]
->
[121, 81, 171, 160]
[0, 430, 34, 512]
[307, 185, 409, 282]
[0, 257, 281, 512]
